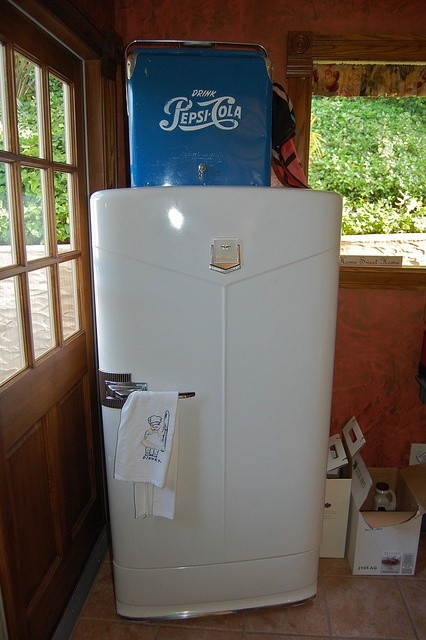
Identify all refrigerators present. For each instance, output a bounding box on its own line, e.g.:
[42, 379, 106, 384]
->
[89, 186, 343, 621]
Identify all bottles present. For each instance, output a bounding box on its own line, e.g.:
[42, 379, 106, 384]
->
[373, 482, 397, 511]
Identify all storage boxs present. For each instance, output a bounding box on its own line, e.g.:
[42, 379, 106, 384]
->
[320, 416, 365, 558]
[125, 40, 272, 186]
[346, 451, 426, 576]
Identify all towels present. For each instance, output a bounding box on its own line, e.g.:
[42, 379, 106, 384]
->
[114, 391, 180, 520]
